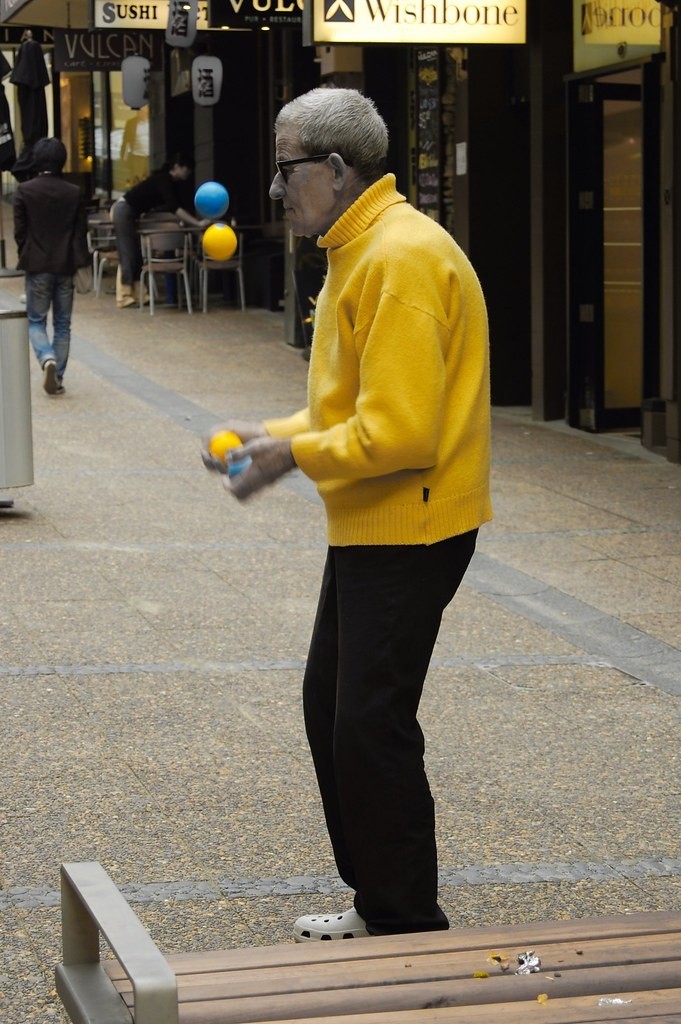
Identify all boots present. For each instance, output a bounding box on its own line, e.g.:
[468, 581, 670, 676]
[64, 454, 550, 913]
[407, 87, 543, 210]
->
[132, 281, 152, 305]
[117, 284, 137, 308]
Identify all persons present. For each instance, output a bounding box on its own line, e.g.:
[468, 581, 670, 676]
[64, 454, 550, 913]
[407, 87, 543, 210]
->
[120, 105, 149, 176]
[199, 86, 495, 944]
[10, 137, 88, 396]
[113, 151, 211, 310]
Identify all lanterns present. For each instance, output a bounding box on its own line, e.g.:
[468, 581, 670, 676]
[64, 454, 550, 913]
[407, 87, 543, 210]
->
[161, 0, 198, 49]
[190, 54, 223, 109]
[120, 56, 151, 113]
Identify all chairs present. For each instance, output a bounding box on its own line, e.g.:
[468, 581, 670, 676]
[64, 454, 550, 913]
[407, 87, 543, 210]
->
[84, 198, 264, 313]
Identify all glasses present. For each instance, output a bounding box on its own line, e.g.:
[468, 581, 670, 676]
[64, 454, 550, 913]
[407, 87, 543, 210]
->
[274, 150, 355, 182]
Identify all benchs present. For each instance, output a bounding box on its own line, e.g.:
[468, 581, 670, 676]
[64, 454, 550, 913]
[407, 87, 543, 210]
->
[53, 857, 681, 1023]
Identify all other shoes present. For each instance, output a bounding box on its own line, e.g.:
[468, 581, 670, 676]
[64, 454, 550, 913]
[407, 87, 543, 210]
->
[42, 357, 59, 396]
[53, 386, 66, 392]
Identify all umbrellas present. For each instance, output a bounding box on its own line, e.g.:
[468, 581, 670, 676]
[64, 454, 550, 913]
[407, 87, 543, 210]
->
[0, 39, 51, 270]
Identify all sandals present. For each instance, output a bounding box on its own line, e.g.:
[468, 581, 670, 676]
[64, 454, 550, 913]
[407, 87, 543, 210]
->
[292, 906, 372, 943]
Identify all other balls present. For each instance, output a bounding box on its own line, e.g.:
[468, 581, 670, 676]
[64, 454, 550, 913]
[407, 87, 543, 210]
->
[209, 430, 253, 478]
[193, 181, 239, 262]
